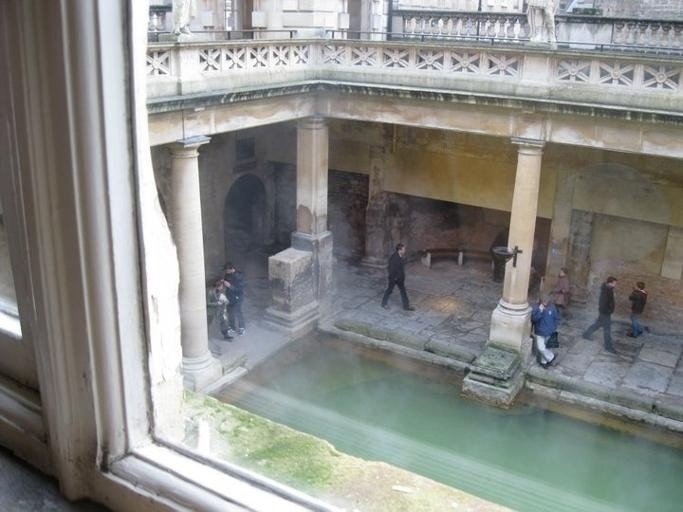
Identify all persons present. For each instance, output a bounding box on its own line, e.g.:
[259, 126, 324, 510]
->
[552, 267, 572, 311]
[529, 294, 561, 369]
[581, 276, 620, 354]
[380, 243, 416, 311]
[625, 281, 648, 338]
[490, 227, 508, 283]
[206, 261, 247, 340]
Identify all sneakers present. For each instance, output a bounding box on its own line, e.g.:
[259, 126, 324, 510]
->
[540, 352, 557, 369]
[583, 334, 593, 341]
[382, 303, 391, 310]
[604, 346, 616, 353]
[627, 329, 643, 338]
[223, 328, 247, 341]
[403, 306, 415, 311]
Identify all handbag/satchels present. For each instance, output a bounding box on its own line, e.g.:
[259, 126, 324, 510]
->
[546, 331, 560, 349]
